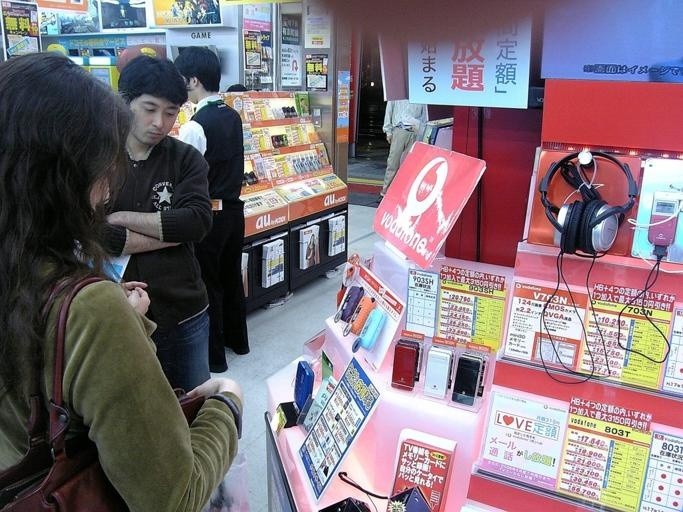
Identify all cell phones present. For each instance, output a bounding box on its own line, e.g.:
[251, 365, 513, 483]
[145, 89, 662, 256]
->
[650, 193, 678, 245]
[391, 339, 483, 406]
[337, 286, 386, 350]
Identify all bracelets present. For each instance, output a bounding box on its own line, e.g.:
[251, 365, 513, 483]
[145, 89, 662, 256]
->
[207, 394, 242, 439]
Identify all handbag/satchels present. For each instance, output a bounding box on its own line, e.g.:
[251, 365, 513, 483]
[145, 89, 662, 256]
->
[0, 269, 205, 512]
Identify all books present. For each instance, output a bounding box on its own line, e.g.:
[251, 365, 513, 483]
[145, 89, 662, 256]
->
[261, 215, 345, 288]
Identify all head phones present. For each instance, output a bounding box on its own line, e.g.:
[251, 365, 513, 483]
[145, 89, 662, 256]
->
[539, 151, 639, 255]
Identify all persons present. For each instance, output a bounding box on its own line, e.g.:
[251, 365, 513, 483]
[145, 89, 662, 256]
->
[1, 52, 243, 510]
[376, 100, 429, 203]
[81, 47, 248, 397]
